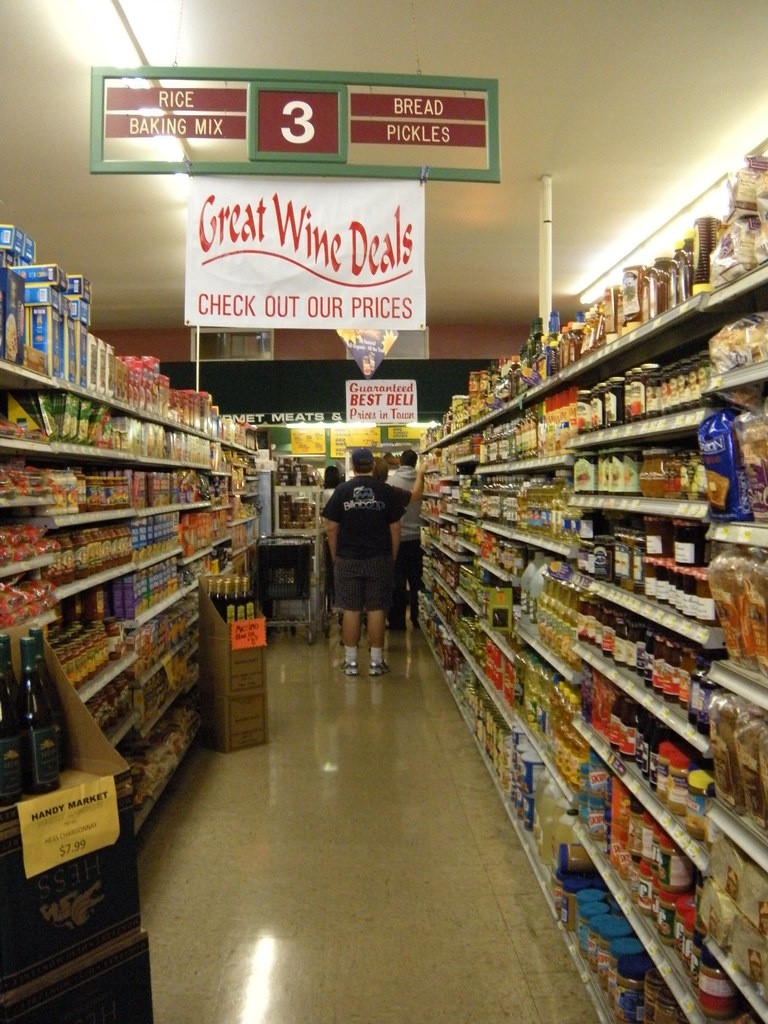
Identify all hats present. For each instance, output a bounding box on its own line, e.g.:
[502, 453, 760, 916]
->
[351, 448, 373, 466]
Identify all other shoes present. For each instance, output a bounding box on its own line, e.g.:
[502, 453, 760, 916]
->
[390, 624, 407, 631]
[411, 616, 421, 628]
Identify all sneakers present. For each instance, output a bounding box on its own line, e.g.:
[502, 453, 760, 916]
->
[369, 661, 390, 674]
[339, 659, 360, 674]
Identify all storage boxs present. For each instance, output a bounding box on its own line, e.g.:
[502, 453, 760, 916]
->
[199, 592, 268, 753]
[0, 622, 153, 1024]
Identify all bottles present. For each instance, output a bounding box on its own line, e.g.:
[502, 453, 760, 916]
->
[692, 217, 717, 295]
[697, 946, 737, 1018]
[690, 656, 711, 724]
[39, 523, 134, 589]
[698, 571, 720, 628]
[450, 230, 697, 1024]
[698, 350, 717, 407]
[36, 467, 130, 516]
[690, 927, 707, 986]
[698, 663, 721, 735]
[0, 620, 132, 807]
[207, 577, 255, 623]
[685, 769, 716, 841]
[84, 585, 106, 621]
[62, 594, 82, 620]
[691, 460, 709, 499]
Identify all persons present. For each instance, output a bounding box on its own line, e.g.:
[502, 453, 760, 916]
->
[386, 450, 422, 632]
[320, 448, 407, 675]
[372, 457, 389, 482]
[322, 466, 340, 612]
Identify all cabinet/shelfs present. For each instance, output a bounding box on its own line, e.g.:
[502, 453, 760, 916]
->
[411, 260, 768, 1024]
[0, 357, 259, 853]
